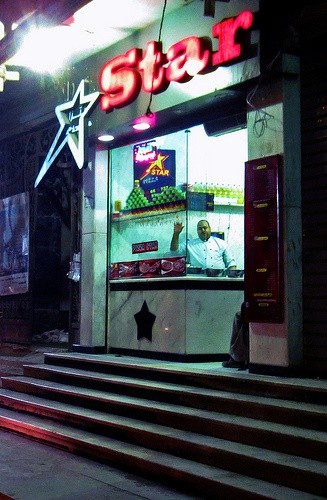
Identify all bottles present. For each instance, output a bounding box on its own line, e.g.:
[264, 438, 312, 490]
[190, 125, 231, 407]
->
[193, 182, 244, 205]
[123, 180, 186, 211]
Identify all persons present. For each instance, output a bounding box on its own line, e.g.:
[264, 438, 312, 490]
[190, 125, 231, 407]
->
[222, 310, 250, 371]
[169, 220, 238, 271]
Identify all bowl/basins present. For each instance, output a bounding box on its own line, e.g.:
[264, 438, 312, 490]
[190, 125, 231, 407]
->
[187, 267, 202, 273]
[225, 270, 244, 278]
[201, 268, 227, 277]
